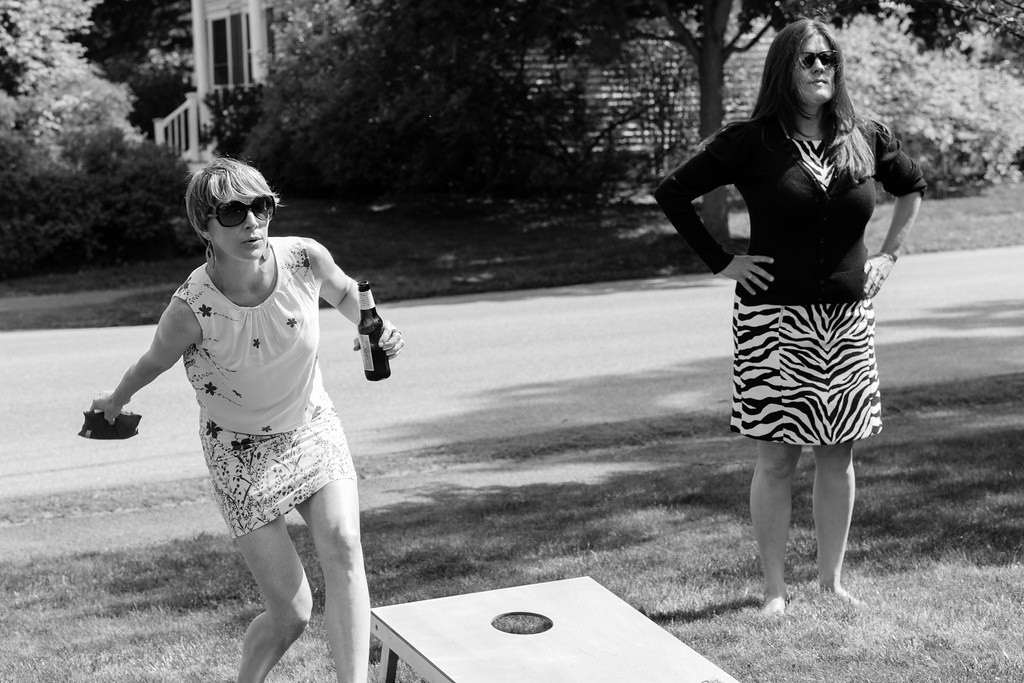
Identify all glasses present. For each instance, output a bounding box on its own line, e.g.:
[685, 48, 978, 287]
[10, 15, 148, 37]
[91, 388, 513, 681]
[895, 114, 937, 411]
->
[206, 193, 276, 227]
[798, 49, 839, 69]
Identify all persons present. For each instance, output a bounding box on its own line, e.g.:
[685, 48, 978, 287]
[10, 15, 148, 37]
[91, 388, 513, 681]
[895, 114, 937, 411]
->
[653, 19, 927, 615]
[89, 159, 405, 683]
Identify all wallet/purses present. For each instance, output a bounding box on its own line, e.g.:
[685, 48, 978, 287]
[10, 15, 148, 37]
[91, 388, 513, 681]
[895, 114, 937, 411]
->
[77, 409, 142, 440]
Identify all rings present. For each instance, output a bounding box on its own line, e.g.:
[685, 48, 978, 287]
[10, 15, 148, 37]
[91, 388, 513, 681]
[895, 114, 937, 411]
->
[391, 328, 401, 336]
[744, 269, 751, 279]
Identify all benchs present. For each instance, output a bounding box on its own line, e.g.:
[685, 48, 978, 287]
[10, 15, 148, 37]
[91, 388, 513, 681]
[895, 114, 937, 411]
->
[369, 577, 741, 683]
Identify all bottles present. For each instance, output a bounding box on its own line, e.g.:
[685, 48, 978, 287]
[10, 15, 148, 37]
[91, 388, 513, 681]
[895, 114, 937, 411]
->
[356, 280, 391, 382]
